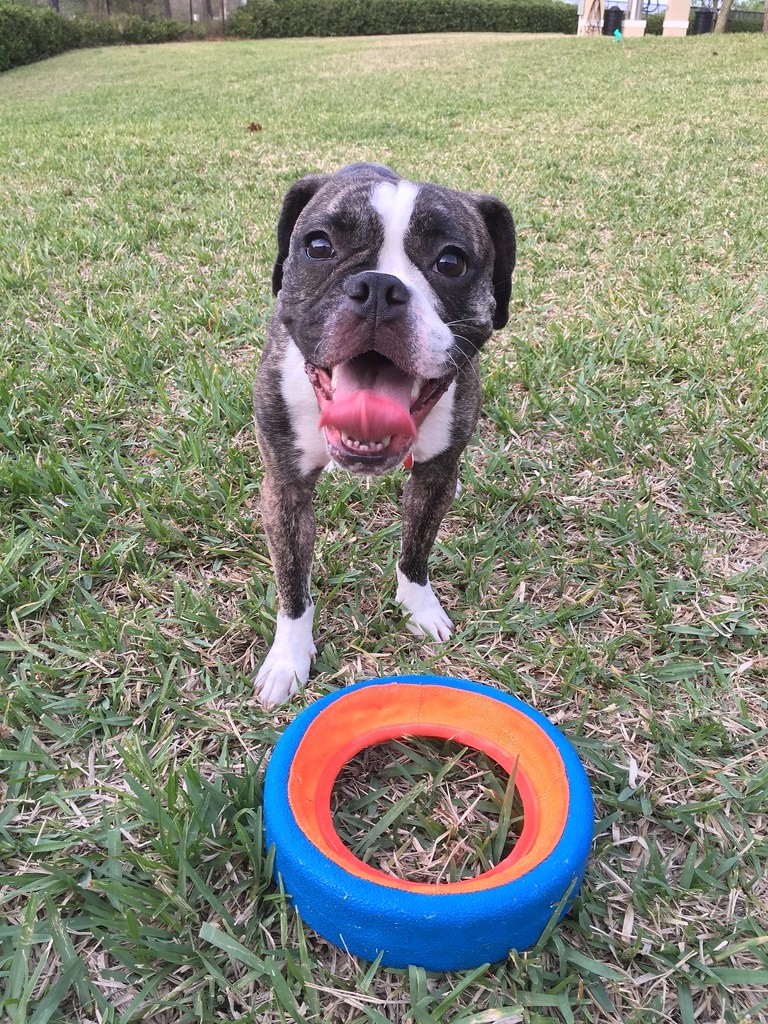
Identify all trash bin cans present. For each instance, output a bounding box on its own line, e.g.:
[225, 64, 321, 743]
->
[604, 6, 624, 35]
[692, 7, 715, 35]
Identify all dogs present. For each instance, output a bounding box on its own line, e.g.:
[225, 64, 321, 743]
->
[250, 161, 518, 716]
